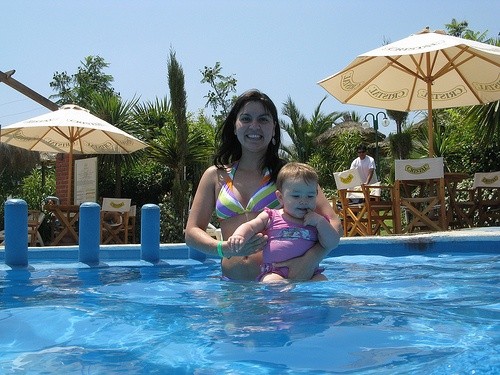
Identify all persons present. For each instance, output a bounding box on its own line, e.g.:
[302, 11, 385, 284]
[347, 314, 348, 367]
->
[229, 162, 340, 285]
[185, 88, 343, 281]
[350, 144, 378, 203]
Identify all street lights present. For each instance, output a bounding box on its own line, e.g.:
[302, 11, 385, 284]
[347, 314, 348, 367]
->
[362, 111, 390, 200]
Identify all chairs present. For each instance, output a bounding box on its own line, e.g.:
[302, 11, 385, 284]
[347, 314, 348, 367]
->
[26, 157, 500, 247]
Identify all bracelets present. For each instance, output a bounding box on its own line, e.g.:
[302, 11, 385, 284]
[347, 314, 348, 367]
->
[217, 241, 231, 259]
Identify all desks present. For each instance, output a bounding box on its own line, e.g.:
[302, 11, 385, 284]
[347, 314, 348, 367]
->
[403, 173, 472, 233]
[43, 205, 80, 247]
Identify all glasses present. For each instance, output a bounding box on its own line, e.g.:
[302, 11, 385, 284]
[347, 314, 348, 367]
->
[358, 151, 365, 153]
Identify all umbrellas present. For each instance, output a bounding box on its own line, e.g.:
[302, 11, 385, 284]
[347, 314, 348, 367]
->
[316, 26, 500, 189]
[1, 103, 149, 221]
